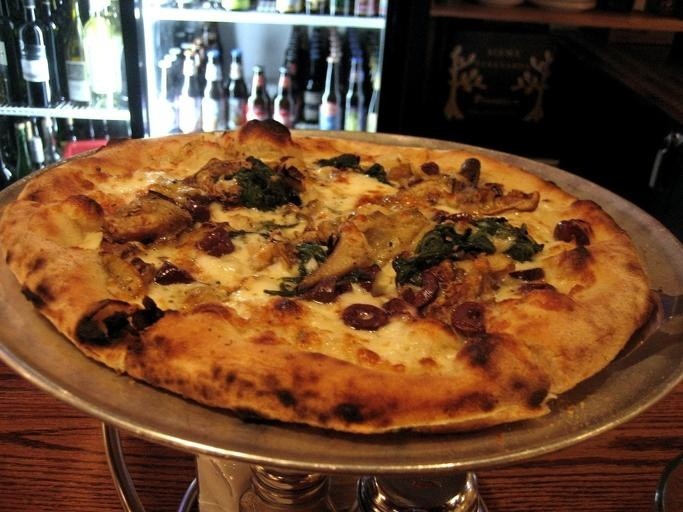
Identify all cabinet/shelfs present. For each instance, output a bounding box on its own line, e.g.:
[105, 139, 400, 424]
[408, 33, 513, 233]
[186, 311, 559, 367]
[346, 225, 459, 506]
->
[556, 41, 681, 243]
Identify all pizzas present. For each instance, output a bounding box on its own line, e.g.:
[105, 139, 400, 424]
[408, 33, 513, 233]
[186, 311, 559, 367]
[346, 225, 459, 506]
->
[0, 119, 654, 436]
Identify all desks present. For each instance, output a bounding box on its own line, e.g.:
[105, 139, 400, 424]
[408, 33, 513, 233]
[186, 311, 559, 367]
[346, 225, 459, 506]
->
[0, 353, 682, 509]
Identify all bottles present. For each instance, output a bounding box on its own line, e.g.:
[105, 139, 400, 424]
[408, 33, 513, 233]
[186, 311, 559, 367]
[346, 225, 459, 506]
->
[153, 1, 385, 139]
[239, 462, 491, 512]
[1, 0, 135, 175]
